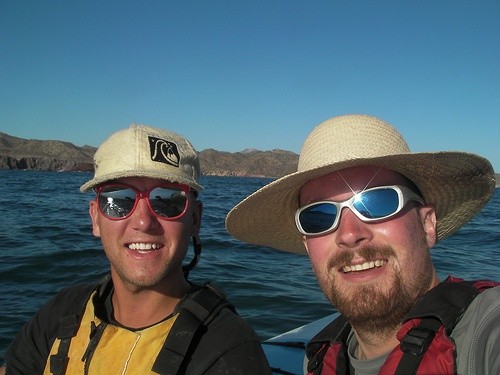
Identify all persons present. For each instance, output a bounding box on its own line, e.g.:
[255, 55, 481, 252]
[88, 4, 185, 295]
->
[225, 114, 500, 375]
[0, 125, 270, 375]
